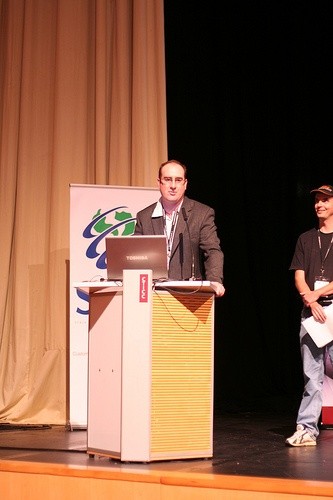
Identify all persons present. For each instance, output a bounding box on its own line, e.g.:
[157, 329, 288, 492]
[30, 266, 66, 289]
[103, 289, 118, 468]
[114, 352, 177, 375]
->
[132, 160, 226, 298]
[286, 183, 333, 447]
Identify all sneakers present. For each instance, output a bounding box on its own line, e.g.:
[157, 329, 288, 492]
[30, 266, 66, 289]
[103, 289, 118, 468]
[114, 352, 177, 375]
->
[285, 423, 317, 447]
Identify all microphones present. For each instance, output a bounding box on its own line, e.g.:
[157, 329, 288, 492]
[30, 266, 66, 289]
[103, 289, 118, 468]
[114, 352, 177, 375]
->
[182, 206, 196, 281]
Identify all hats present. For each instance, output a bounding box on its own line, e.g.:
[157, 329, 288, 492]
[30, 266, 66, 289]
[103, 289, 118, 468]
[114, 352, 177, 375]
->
[309, 185, 333, 196]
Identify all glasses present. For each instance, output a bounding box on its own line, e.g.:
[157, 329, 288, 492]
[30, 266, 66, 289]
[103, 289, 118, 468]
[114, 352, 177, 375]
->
[160, 178, 186, 184]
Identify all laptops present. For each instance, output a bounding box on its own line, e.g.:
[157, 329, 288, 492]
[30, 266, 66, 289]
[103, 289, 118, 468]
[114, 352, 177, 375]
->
[105, 235, 168, 282]
